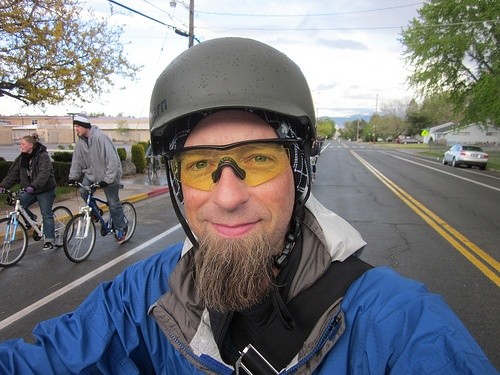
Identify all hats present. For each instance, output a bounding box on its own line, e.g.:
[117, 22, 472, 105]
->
[72, 110, 92, 128]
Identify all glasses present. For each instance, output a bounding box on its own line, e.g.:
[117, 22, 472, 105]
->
[163, 136, 302, 193]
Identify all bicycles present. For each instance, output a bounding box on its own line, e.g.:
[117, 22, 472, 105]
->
[62, 183, 137, 263]
[145, 156, 162, 181]
[0, 189, 75, 268]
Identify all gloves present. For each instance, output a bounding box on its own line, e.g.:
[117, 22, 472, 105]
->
[26, 187, 34, 194]
[68, 179, 75, 184]
[0, 187, 5, 193]
[98, 181, 108, 188]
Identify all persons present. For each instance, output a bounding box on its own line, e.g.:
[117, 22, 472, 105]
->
[0, 37, 500, 375]
[69, 113, 127, 243]
[0, 133, 56, 250]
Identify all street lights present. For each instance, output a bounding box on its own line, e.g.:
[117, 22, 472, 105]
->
[170, 0, 194, 49]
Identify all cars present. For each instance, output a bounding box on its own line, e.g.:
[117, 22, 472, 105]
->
[398, 136, 421, 145]
[443, 144, 489, 170]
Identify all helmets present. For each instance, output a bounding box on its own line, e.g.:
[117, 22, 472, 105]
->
[149, 36, 317, 156]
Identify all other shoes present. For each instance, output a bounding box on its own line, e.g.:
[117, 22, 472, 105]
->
[117, 229, 126, 244]
[26, 214, 37, 227]
[43, 242, 54, 250]
[89, 209, 103, 221]
